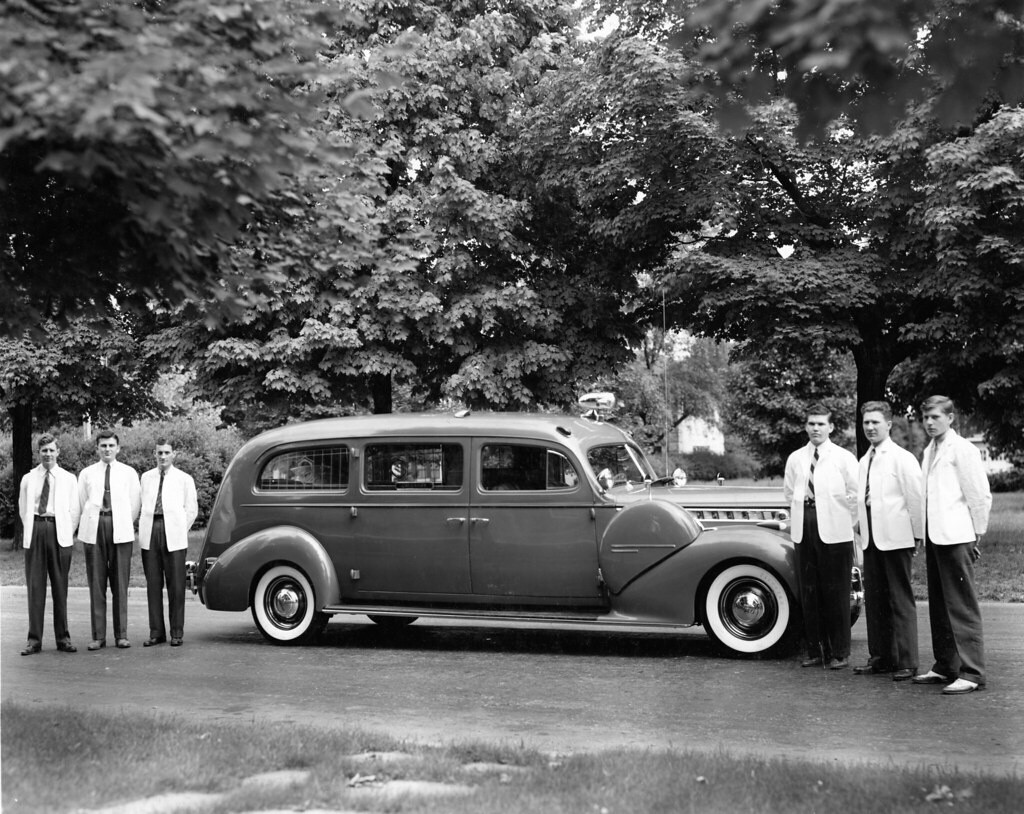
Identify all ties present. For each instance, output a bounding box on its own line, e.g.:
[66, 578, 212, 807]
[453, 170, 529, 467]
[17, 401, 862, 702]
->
[802, 444, 819, 508]
[864, 449, 876, 505]
[103, 464, 111, 508]
[154, 470, 165, 514]
[37, 470, 50, 515]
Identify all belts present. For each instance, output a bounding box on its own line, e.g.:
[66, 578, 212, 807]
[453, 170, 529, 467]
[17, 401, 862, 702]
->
[34, 515, 55, 522]
[154, 514, 163, 518]
[100, 511, 112, 516]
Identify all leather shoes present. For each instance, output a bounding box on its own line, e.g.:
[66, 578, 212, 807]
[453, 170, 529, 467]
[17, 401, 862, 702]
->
[911, 671, 950, 683]
[170, 637, 183, 646]
[57, 644, 76, 652]
[893, 667, 918, 680]
[143, 637, 166, 647]
[20, 644, 41, 655]
[854, 665, 888, 673]
[942, 678, 985, 694]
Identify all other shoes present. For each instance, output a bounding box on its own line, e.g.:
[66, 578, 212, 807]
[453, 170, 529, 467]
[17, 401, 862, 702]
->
[828, 654, 848, 669]
[88, 640, 106, 649]
[801, 656, 823, 667]
[115, 638, 131, 648]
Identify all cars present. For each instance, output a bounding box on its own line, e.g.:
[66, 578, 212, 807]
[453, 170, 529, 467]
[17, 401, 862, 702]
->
[184, 414, 865, 661]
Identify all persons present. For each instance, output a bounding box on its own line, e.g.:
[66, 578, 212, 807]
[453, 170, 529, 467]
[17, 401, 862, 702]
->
[19, 434, 80, 655]
[910, 395, 993, 695]
[783, 402, 859, 669]
[853, 401, 923, 681]
[140, 434, 198, 647]
[78, 430, 140, 651]
